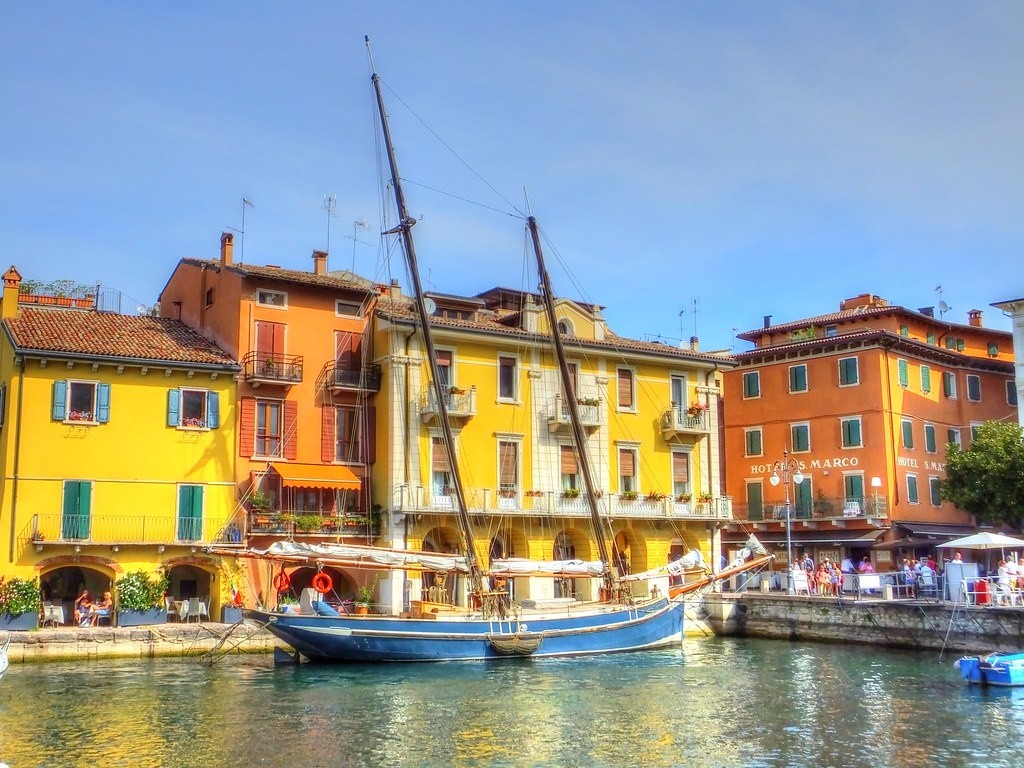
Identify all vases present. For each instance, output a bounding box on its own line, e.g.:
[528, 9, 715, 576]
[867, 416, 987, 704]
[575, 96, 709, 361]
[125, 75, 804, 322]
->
[34, 295, 56, 304]
[74, 298, 94, 307]
[354, 605, 369, 615]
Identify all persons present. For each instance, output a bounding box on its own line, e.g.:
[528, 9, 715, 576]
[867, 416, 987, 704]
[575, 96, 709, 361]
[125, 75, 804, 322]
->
[87, 592, 112, 628]
[791, 551, 1024, 607]
[651, 585, 661, 599]
[40, 576, 55, 605]
[73, 590, 94, 628]
[668, 553, 683, 586]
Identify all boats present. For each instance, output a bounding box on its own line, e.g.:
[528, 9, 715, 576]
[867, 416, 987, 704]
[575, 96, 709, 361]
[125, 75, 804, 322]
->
[953, 649, 1024, 686]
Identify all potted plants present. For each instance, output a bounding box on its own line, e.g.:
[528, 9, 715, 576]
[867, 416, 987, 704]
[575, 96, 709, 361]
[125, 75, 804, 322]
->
[564, 487, 579, 498]
[321, 518, 369, 526]
[113, 569, 170, 626]
[499, 488, 518, 498]
[584, 398, 600, 406]
[17, 281, 36, 303]
[619, 491, 711, 503]
[0, 576, 43, 630]
[448, 386, 464, 394]
[261, 359, 275, 376]
[53, 291, 74, 306]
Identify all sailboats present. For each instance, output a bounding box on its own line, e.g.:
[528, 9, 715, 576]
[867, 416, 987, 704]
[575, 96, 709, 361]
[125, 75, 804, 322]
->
[202, 36, 776, 668]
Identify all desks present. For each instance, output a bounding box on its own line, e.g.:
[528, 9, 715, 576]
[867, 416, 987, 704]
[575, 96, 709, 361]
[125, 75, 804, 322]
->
[172, 601, 207, 622]
[44, 606, 64, 626]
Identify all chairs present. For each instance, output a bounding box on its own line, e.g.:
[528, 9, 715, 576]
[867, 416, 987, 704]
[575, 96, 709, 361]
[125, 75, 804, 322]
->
[96, 602, 114, 627]
[164, 596, 179, 622]
[40, 601, 60, 628]
[186, 597, 200, 623]
[200, 596, 211, 622]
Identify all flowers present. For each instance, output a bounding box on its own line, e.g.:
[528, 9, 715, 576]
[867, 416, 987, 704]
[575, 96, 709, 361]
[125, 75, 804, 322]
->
[182, 417, 204, 427]
[69, 409, 92, 420]
[686, 403, 709, 417]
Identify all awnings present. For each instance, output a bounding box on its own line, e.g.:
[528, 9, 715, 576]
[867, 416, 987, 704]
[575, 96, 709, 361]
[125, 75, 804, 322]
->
[721, 530, 886, 543]
[894, 519, 983, 535]
[269, 460, 363, 490]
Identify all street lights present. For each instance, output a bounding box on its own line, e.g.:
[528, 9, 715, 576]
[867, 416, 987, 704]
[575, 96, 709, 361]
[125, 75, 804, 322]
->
[768, 443, 803, 595]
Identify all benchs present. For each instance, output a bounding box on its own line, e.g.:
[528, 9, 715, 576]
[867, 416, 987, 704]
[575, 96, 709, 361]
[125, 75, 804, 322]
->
[882, 583, 1024, 605]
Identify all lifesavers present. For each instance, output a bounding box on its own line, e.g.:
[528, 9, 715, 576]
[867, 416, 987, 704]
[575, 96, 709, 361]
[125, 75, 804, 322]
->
[312, 573, 333, 594]
[273, 573, 291, 591]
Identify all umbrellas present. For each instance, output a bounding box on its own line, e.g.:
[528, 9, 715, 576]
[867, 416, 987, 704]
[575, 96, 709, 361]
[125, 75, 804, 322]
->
[934, 532, 1024, 576]
